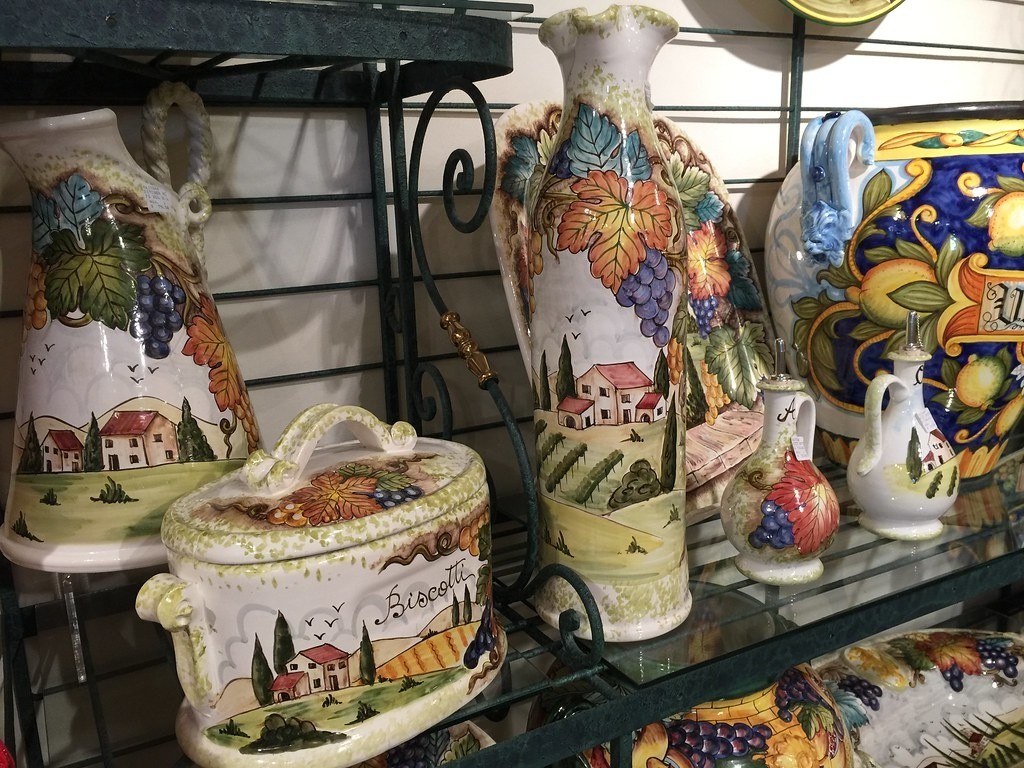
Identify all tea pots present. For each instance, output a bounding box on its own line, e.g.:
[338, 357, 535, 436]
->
[720, 339, 838, 586]
[847, 311, 959, 540]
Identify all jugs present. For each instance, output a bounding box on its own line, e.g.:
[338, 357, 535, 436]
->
[1, 82, 263, 573]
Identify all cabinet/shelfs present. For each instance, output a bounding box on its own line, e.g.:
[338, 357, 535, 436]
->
[0, 0, 1024, 768]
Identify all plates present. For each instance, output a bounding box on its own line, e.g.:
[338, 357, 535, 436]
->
[812, 628, 1024, 768]
[484, 101, 787, 562]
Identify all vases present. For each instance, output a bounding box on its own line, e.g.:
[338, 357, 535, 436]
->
[526, 5, 694, 642]
